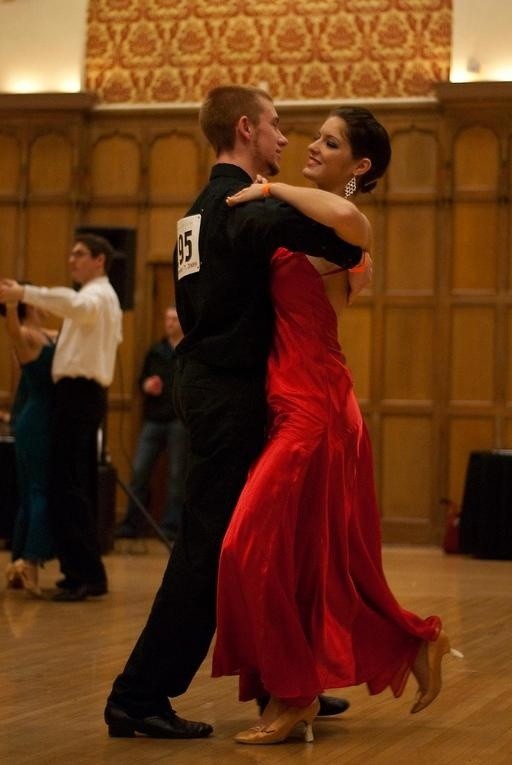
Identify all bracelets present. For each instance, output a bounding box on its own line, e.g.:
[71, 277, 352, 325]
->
[262, 183, 275, 197]
[348, 251, 370, 273]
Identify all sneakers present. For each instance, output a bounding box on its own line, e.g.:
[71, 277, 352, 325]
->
[54, 576, 106, 601]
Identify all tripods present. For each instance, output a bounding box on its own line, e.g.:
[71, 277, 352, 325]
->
[101, 434, 172, 553]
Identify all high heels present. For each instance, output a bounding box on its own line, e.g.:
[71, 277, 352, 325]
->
[234, 697, 320, 744]
[410, 628, 463, 714]
[7, 560, 44, 601]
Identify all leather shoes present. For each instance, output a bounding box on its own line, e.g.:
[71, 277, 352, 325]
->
[104, 700, 213, 738]
[257, 693, 349, 716]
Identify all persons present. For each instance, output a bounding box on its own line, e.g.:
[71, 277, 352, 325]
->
[0, 282, 60, 599]
[210, 109, 463, 746]
[104, 84, 375, 740]
[112, 304, 186, 541]
[0, 235, 123, 601]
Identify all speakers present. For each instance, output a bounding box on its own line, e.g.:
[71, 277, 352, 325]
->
[460, 449, 512, 560]
[74, 226, 137, 309]
